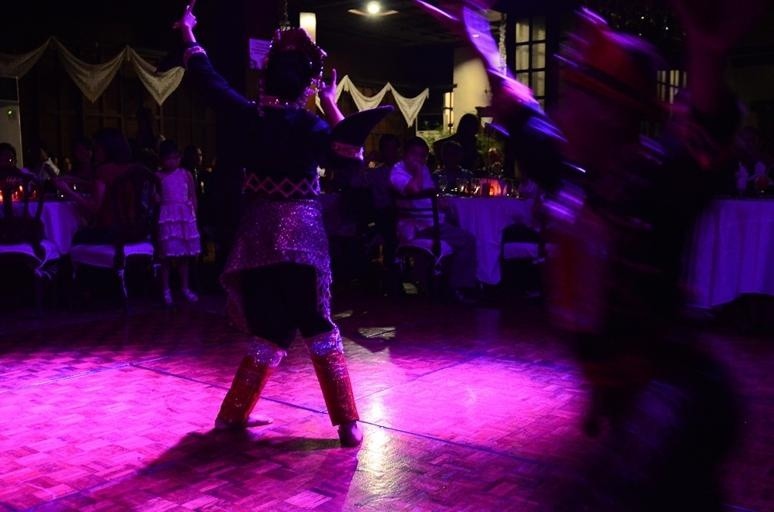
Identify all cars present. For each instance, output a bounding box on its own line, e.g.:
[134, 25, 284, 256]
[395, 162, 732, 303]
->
[438, 173, 481, 197]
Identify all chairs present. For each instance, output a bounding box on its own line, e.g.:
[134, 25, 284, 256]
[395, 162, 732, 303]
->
[160, 285, 174, 306]
[180, 285, 199, 304]
[1, 158, 774, 314]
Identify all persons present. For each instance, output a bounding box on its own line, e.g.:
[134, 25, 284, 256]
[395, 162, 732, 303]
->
[173, 2, 364, 452]
[459, 1, 755, 510]
[724, 101, 771, 197]
[0, 133, 247, 310]
[332, 112, 553, 300]
